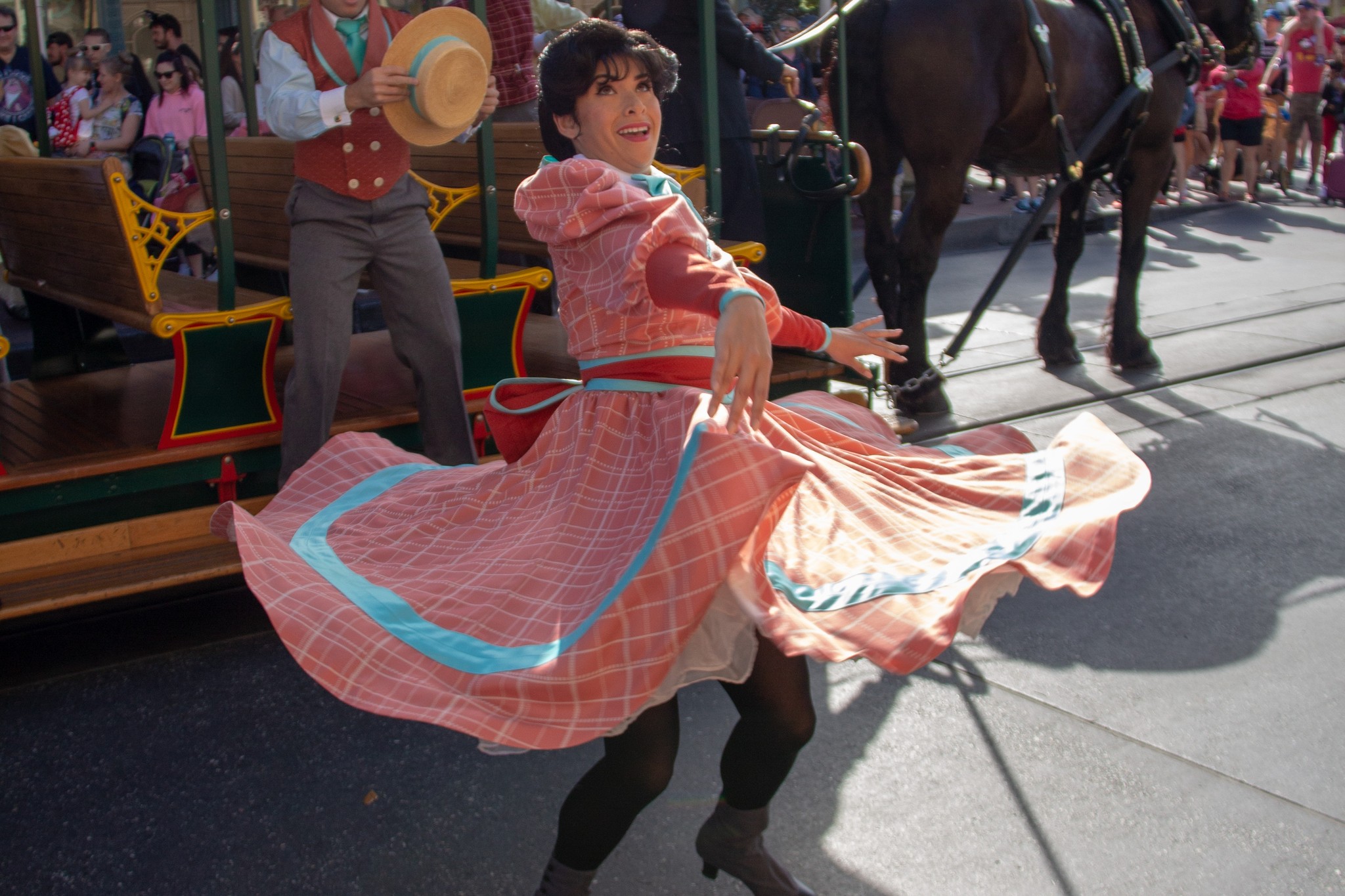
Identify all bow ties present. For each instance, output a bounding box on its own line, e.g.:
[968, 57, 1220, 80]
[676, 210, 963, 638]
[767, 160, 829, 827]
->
[631, 173, 704, 222]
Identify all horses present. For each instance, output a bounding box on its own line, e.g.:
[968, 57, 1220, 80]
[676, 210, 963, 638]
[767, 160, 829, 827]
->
[821, 0, 1262, 413]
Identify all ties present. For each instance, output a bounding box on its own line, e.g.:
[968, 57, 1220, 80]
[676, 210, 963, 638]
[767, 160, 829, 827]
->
[335, 18, 366, 78]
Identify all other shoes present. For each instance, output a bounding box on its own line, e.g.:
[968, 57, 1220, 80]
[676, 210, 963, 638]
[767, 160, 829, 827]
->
[1031, 197, 1043, 211]
[1153, 155, 1320, 210]
[1014, 199, 1031, 212]
[1278, 59, 1288, 68]
[1000, 185, 1016, 203]
[1314, 54, 1324, 65]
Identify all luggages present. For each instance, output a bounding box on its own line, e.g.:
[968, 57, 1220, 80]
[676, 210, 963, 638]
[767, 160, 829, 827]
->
[1323, 126, 1345, 204]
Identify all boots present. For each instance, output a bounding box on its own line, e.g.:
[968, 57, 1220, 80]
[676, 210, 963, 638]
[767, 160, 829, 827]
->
[532, 857, 599, 895]
[695, 793, 815, 896]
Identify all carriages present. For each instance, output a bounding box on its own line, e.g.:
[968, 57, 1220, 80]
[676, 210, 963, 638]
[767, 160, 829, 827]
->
[0, 1, 1264, 618]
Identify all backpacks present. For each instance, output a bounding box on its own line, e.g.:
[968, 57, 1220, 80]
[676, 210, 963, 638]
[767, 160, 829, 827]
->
[45, 84, 87, 149]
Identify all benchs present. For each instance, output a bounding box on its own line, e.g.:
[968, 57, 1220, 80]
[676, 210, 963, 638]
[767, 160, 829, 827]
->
[0, 156, 293, 450]
[192, 137, 553, 402]
[407, 123, 768, 273]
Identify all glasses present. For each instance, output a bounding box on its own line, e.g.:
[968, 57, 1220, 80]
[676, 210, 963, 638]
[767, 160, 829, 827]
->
[0, 25, 15, 32]
[778, 25, 800, 33]
[81, 44, 109, 51]
[153, 67, 179, 80]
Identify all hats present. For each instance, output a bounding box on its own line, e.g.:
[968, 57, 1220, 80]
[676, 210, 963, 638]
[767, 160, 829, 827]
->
[1295, 0, 1313, 10]
[380, 7, 493, 147]
[1263, 9, 1281, 21]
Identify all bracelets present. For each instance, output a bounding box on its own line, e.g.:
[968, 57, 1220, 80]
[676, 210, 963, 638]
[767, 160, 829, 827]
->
[90, 140, 95, 151]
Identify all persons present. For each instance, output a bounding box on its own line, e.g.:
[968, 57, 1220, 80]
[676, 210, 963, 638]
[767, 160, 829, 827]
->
[1112, 0, 1345, 210]
[260, 0, 499, 491]
[209, 21, 1151, 896]
[743, 11, 818, 101]
[442, 0, 800, 303]
[1012, 177, 1043, 212]
[0, 3, 265, 278]
